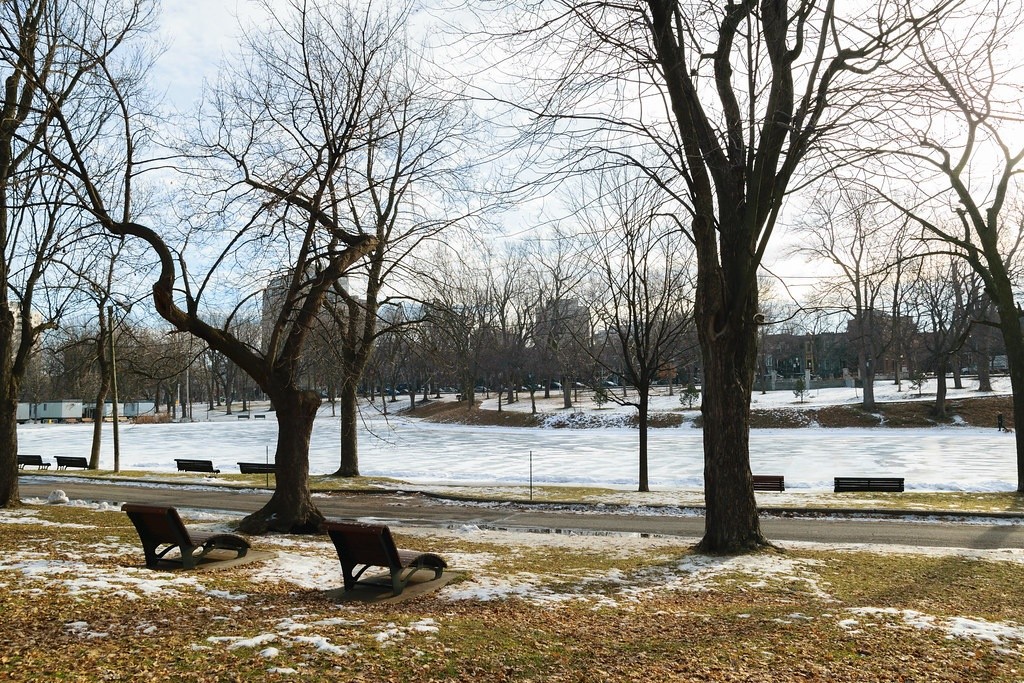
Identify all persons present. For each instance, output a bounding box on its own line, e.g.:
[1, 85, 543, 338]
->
[996, 411, 1007, 431]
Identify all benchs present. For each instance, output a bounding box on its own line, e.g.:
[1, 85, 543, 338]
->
[238, 416, 250, 420]
[17, 455, 51, 470]
[238, 462, 275, 474]
[255, 415, 265, 418]
[752, 475, 786, 493]
[54, 456, 89, 470]
[174, 458, 220, 474]
[834, 477, 905, 492]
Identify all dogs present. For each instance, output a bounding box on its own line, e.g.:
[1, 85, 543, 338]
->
[1002, 428, 1014, 433]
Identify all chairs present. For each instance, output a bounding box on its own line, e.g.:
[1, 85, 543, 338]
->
[121, 504, 252, 569]
[325, 518, 448, 595]
